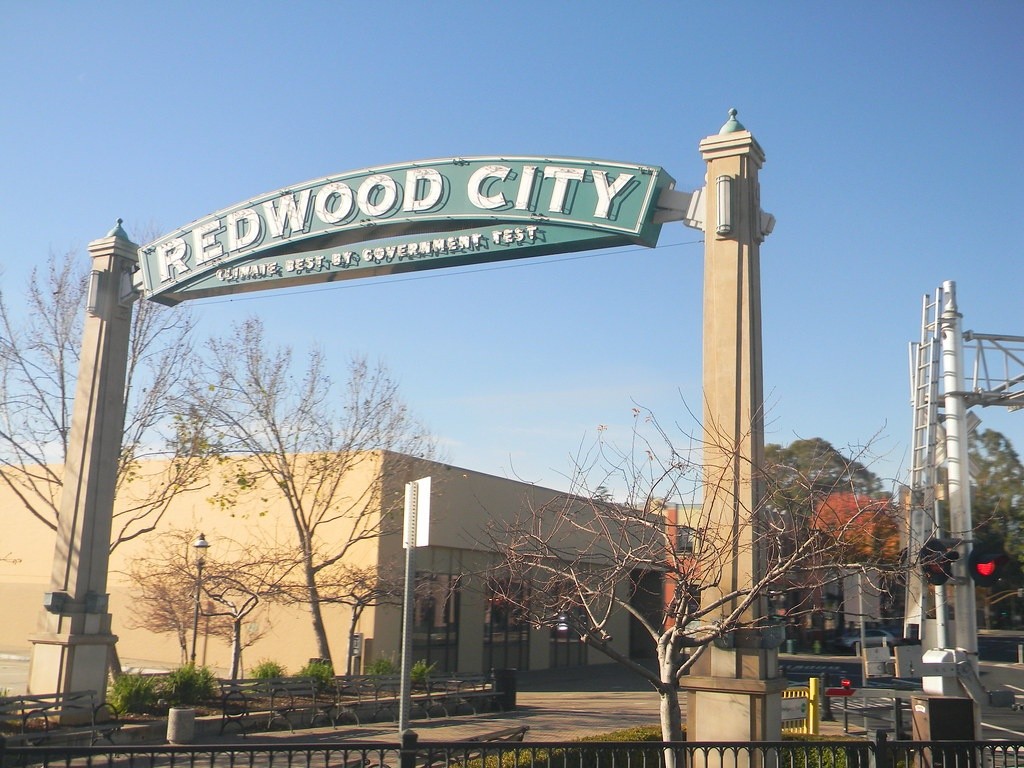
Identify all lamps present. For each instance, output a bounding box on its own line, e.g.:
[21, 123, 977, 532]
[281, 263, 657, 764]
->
[714, 174, 734, 234]
[86, 269, 103, 317]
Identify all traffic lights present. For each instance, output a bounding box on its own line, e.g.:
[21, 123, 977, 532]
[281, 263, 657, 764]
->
[919, 535, 960, 586]
[839, 679, 851, 689]
[970, 551, 1007, 589]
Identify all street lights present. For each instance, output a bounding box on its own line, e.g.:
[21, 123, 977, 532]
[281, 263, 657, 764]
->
[188, 532, 210, 668]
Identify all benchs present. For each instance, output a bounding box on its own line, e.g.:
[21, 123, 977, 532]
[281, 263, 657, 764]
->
[217, 676, 338, 740]
[332, 672, 430, 728]
[428, 670, 508, 719]
[0, 689, 125, 768]
